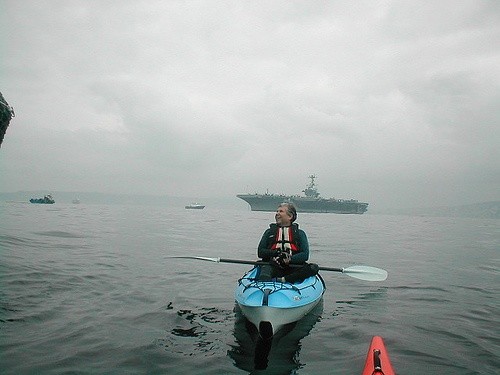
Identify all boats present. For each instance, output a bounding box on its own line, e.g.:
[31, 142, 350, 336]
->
[185, 202, 205, 210]
[30, 195, 56, 204]
[234, 262, 325, 341]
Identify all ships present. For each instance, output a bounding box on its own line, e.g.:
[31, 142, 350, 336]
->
[235, 174, 370, 216]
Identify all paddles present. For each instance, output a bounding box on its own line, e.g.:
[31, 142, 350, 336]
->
[162, 254, 389, 282]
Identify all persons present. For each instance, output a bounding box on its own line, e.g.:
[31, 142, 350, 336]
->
[254, 200, 319, 281]
